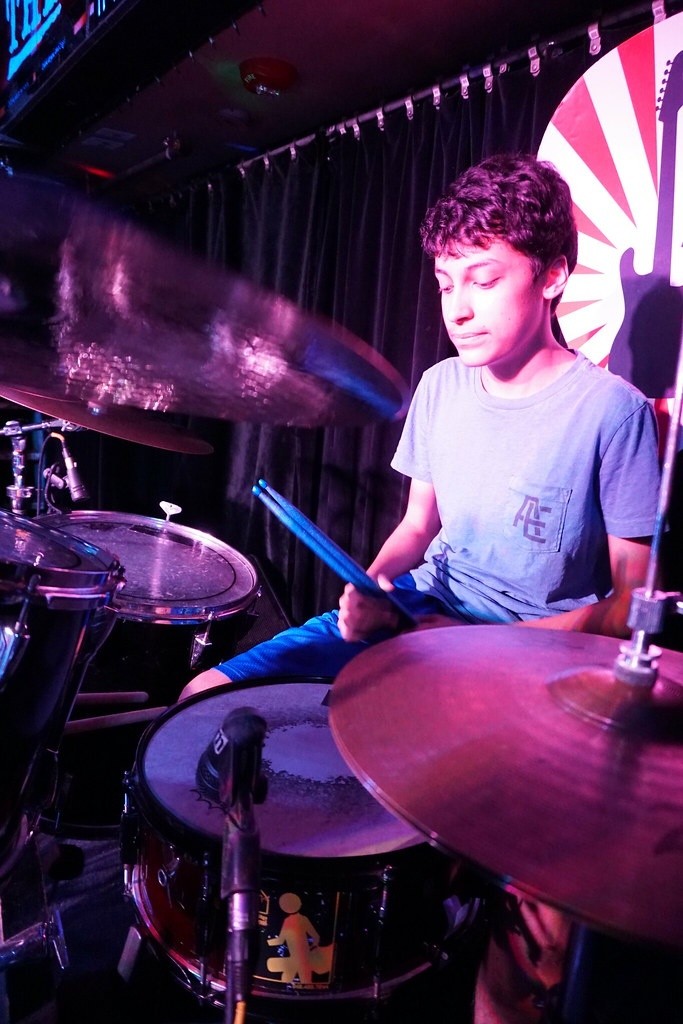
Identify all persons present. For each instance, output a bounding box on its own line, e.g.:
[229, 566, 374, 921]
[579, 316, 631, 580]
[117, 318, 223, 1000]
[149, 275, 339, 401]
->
[178, 150, 658, 1024]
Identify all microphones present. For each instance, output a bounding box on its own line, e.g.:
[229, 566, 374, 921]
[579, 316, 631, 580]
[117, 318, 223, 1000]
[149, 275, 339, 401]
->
[195, 705, 268, 805]
[61, 443, 90, 504]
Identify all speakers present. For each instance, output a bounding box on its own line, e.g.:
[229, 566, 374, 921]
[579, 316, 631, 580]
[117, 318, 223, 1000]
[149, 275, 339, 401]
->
[202, 552, 296, 665]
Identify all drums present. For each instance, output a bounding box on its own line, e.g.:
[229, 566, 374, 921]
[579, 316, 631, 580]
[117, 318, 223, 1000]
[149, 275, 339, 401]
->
[23, 506, 259, 845]
[122, 674, 480, 1024]
[0, 510, 127, 862]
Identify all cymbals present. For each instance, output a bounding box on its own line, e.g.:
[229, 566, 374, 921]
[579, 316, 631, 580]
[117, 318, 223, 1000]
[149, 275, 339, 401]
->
[0, 377, 215, 457]
[325, 624, 682, 953]
